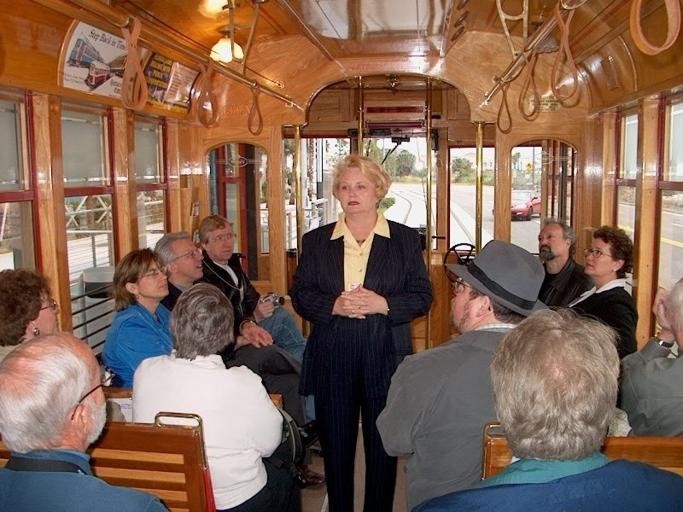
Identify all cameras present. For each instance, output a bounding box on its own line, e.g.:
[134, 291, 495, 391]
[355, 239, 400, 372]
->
[259, 291, 285, 309]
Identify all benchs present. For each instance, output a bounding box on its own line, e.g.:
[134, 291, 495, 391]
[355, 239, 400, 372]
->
[96, 387, 285, 432]
[479, 416, 683, 488]
[0, 407, 216, 511]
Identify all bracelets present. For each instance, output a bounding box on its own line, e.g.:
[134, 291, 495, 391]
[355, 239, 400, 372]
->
[654, 336, 674, 348]
[384, 299, 388, 316]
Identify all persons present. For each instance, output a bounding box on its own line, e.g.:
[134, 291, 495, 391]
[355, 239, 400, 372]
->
[287, 155, 433, 511]
[0, 270, 58, 357]
[132, 284, 301, 512]
[537, 219, 595, 312]
[565, 225, 638, 409]
[375, 240, 549, 512]
[620, 278, 683, 437]
[101, 247, 174, 389]
[158, 214, 325, 488]
[0, 330, 169, 512]
[409, 308, 683, 512]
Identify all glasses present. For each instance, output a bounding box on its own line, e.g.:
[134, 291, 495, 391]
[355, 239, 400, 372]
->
[209, 231, 237, 243]
[143, 266, 168, 279]
[172, 247, 203, 262]
[584, 247, 625, 260]
[39, 300, 57, 312]
[453, 277, 476, 294]
[71, 370, 116, 421]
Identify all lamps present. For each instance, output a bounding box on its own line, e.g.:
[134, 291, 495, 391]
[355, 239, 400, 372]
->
[207, 24, 245, 64]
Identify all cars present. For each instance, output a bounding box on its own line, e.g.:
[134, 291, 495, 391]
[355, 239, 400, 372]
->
[492, 189, 541, 221]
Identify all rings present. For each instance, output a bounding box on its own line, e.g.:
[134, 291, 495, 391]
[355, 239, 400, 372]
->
[359, 306, 361, 310]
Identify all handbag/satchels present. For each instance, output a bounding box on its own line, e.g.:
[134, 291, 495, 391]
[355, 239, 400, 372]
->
[262, 407, 304, 489]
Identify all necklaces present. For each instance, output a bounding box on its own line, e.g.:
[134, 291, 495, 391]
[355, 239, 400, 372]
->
[345, 217, 365, 243]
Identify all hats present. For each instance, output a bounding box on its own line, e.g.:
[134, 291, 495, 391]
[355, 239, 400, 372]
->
[445, 239, 550, 317]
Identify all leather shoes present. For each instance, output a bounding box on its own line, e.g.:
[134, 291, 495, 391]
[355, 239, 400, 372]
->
[301, 464, 326, 490]
